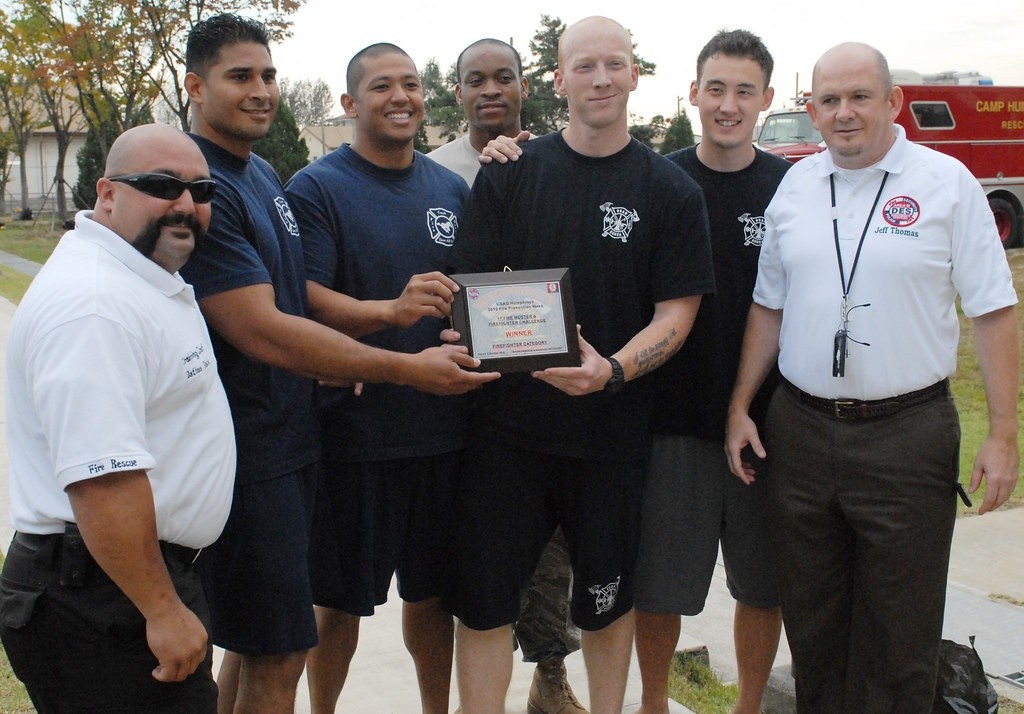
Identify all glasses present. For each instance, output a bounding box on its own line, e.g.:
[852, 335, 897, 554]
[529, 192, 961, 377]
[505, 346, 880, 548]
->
[109, 173, 217, 204]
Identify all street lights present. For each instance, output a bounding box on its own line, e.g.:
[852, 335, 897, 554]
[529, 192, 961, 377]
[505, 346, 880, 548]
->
[678, 95, 683, 116]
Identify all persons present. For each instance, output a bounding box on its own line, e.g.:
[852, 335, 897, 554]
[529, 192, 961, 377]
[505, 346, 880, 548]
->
[0, 123, 236, 714]
[727, 41, 1018, 714]
[284, 43, 470, 714]
[179, 14, 502, 714]
[441, 17, 717, 714]
[425, 37, 590, 714]
[478, 31, 797, 714]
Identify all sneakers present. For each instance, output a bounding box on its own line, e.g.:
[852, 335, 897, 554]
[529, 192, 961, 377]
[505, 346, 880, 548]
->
[527, 666, 590, 714]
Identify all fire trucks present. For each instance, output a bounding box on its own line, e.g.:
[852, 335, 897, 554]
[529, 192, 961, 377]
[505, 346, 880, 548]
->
[749, 70, 1024, 250]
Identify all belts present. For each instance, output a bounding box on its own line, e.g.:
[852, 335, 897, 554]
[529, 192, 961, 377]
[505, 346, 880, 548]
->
[783, 377, 951, 420]
[15, 530, 202, 565]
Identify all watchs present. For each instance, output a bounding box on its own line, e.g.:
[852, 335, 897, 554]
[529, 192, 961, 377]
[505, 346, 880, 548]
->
[604, 355, 626, 397]
